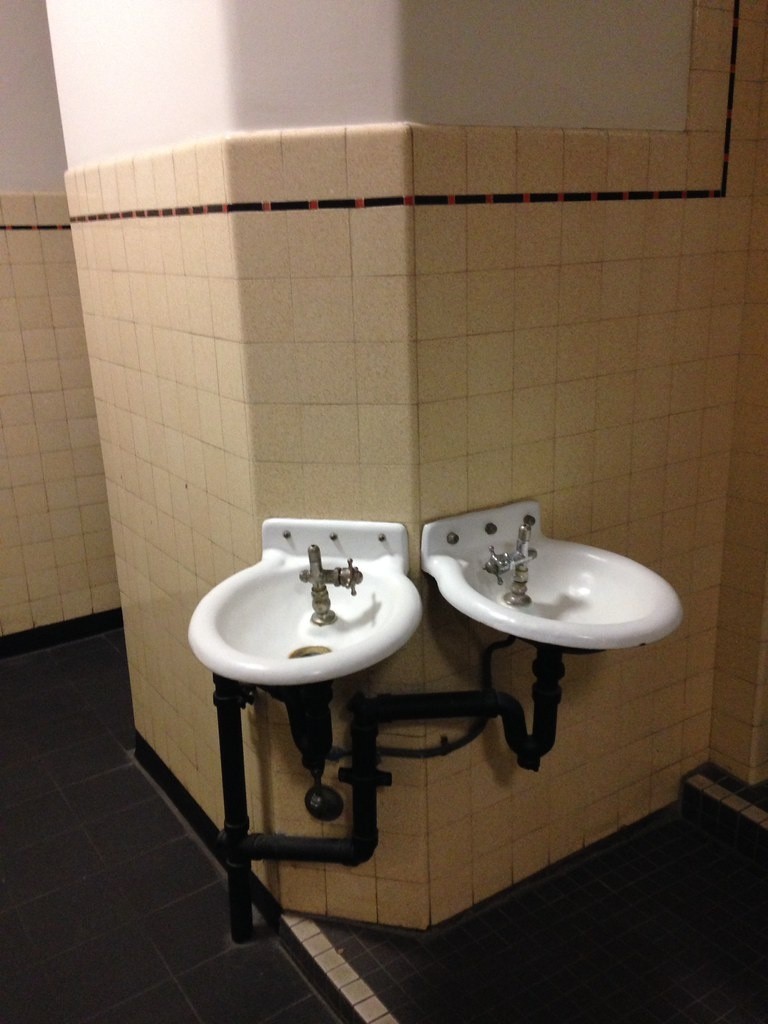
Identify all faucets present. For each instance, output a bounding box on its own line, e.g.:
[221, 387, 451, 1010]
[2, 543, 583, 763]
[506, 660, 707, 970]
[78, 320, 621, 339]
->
[484, 519, 538, 607]
[299, 545, 364, 626]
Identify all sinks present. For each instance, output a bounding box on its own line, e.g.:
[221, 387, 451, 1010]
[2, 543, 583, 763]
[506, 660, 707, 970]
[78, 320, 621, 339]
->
[186, 560, 424, 687]
[436, 536, 684, 651]
